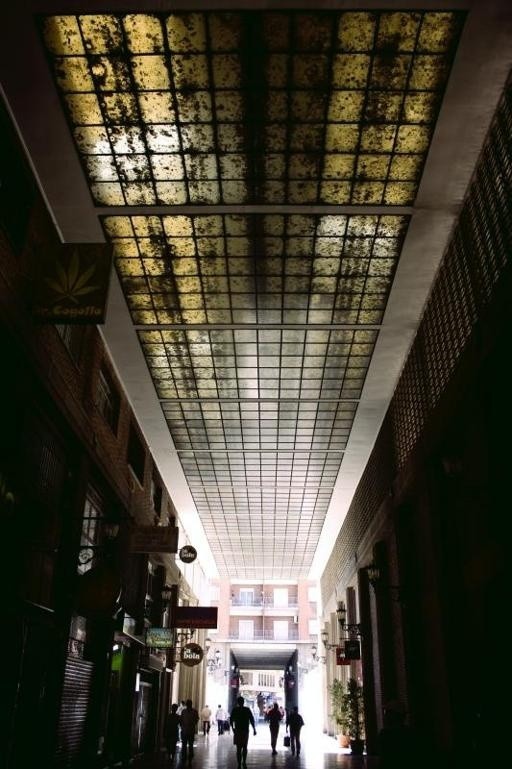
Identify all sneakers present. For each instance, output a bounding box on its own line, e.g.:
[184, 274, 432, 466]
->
[237, 761, 247, 769]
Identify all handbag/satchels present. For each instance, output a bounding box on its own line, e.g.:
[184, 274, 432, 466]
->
[283, 736, 291, 747]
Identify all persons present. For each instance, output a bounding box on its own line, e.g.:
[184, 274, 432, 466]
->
[161, 703, 181, 762]
[216, 705, 225, 735]
[229, 696, 256, 769]
[262, 704, 284, 723]
[265, 704, 284, 755]
[180, 698, 199, 756]
[285, 706, 304, 758]
[176, 700, 186, 743]
[202, 706, 210, 736]
